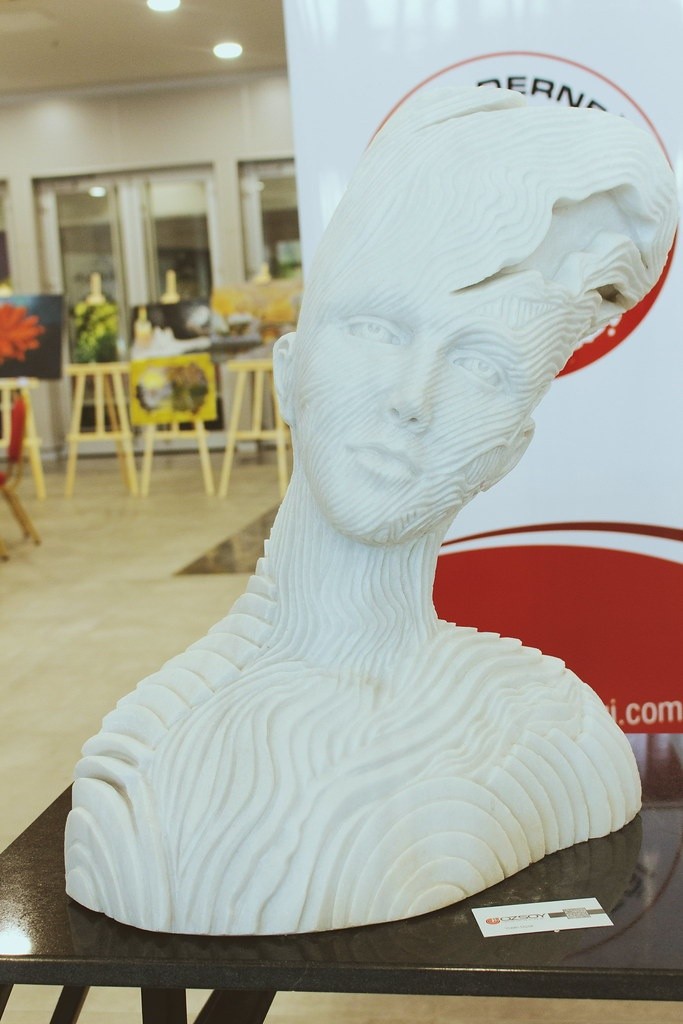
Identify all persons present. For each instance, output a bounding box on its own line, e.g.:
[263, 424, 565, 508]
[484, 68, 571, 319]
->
[63, 85, 678, 938]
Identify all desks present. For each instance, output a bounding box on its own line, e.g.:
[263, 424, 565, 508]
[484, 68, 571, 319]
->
[1, 733, 682, 1023]
